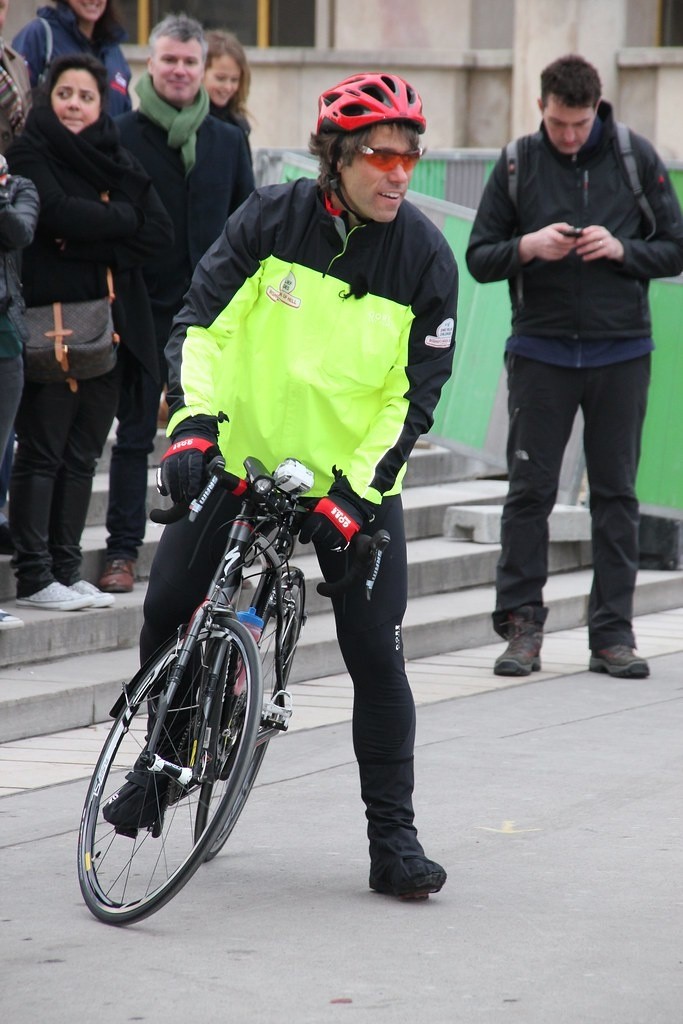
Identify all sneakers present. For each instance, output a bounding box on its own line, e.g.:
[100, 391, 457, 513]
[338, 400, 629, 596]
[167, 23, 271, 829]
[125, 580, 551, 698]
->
[0, 609, 24, 631]
[16, 582, 94, 611]
[492, 605, 548, 676]
[590, 643, 649, 678]
[102, 770, 162, 825]
[370, 856, 447, 899]
[99, 559, 134, 591]
[70, 581, 114, 607]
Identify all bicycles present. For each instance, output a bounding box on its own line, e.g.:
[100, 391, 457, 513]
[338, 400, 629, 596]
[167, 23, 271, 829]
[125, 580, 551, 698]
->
[75, 456, 391, 925]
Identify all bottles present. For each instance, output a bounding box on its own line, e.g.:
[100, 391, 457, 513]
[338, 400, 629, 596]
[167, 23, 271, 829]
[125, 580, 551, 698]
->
[232, 607, 265, 696]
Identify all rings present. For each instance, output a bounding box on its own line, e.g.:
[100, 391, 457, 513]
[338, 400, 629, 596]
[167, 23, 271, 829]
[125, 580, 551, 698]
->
[599, 240, 603, 247]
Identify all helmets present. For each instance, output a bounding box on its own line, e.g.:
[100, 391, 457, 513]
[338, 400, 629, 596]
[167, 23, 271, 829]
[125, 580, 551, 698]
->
[316, 72, 426, 137]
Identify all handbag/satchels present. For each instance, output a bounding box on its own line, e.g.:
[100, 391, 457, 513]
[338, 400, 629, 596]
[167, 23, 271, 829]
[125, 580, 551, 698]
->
[21, 297, 119, 391]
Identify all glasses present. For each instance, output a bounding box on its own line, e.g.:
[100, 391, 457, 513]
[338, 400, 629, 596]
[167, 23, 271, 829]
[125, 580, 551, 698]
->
[355, 145, 422, 171]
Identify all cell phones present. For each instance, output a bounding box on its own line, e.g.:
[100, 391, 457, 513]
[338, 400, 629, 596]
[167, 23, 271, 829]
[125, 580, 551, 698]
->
[564, 228, 583, 238]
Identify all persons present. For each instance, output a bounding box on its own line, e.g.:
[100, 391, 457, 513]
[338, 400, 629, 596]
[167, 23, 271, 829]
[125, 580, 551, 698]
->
[0, 0, 256, 632]
[105, 75, 461, 900]
[466, 53, 683, 678]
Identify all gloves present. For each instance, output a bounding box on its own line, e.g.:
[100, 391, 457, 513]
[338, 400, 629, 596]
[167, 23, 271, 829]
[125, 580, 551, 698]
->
[297, 465, 376, 552]
[156, 411, 229, 504]
